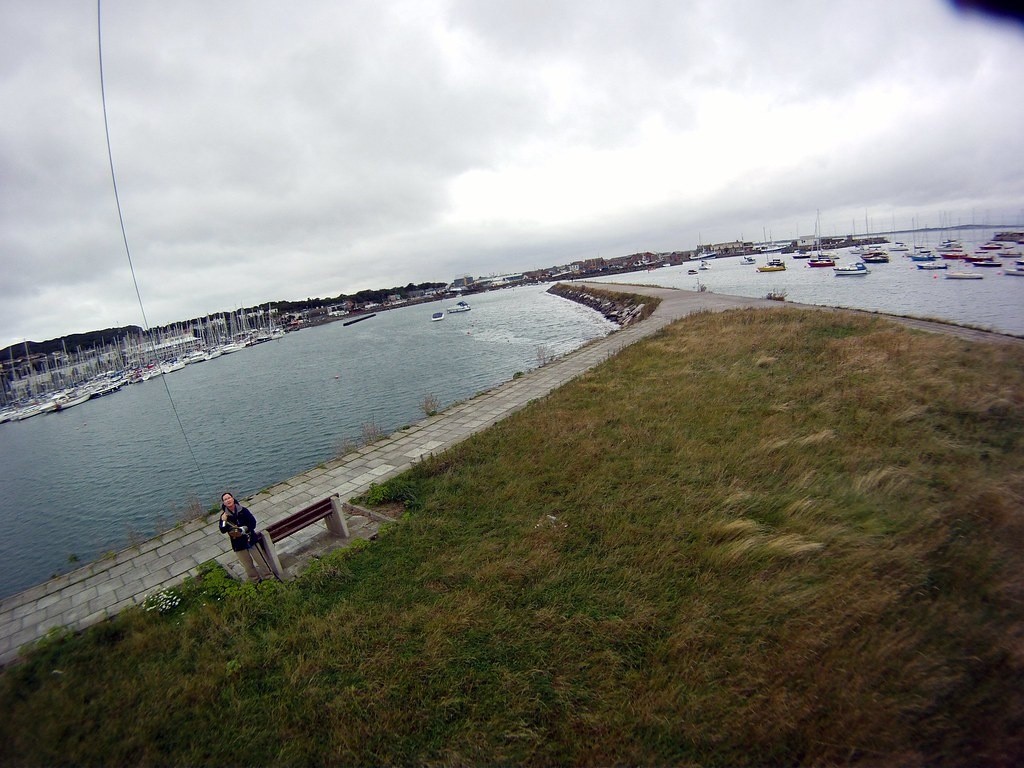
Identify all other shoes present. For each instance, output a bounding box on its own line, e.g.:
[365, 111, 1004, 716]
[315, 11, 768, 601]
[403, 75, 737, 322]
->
[258, 579, 262, 584]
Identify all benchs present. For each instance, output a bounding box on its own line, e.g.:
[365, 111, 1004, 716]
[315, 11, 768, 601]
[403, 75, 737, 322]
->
[257, 492, 349, 577]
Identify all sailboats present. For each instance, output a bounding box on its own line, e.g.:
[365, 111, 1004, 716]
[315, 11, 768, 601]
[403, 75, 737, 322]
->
[0, 272, 286, 426]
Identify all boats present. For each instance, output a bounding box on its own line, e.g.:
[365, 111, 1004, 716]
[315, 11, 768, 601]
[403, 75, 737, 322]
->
[431, 312, 445, 322]
[807, 209, 1024, 276]
[740, 246, 756, 265]
[447, 300, 471, 314]
[755, 226, 786, 273]
[944, 243, 984, 280]
[688, 269, 699, 275]
[698, 253, 711, 270]
[792, 253, 811, 259]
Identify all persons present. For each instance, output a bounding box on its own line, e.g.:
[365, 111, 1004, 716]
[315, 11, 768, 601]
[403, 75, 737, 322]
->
[219, 493, 275, 590]
